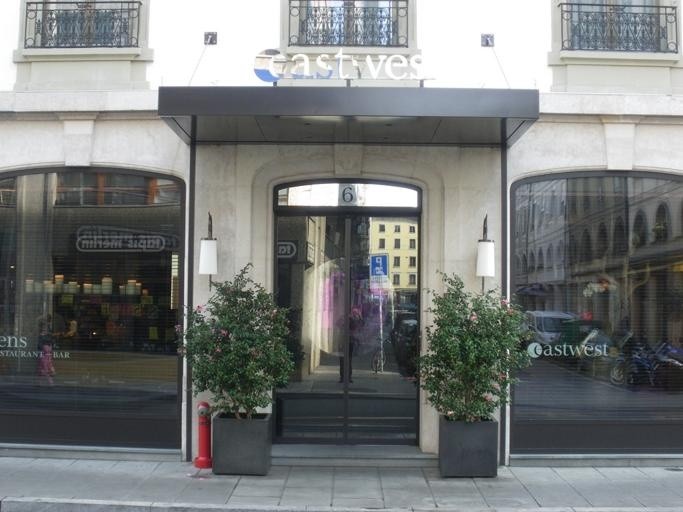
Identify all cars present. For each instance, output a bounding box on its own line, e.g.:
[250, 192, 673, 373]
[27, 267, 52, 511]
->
[387, 302, 417, 374]
[523, 308, 660, 386]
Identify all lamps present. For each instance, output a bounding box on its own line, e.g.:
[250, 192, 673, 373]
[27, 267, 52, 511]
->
[476, 214, 495, 292]
[199, 212, 218, 290]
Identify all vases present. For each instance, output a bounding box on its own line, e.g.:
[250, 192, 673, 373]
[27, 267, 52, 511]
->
[438, 415, 499, 480]
[211, 413, 275, 478]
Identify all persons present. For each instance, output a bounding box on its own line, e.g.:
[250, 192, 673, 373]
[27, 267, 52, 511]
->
[32, 312, 52, 351]
[31, 339, 55, 385]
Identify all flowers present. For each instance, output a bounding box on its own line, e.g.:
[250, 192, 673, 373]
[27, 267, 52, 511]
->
[176, 272, 296, 420]
[404, 268, 535, 422]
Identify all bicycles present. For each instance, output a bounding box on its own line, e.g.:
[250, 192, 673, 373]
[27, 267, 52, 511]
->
[371, 337, 391, 372]
